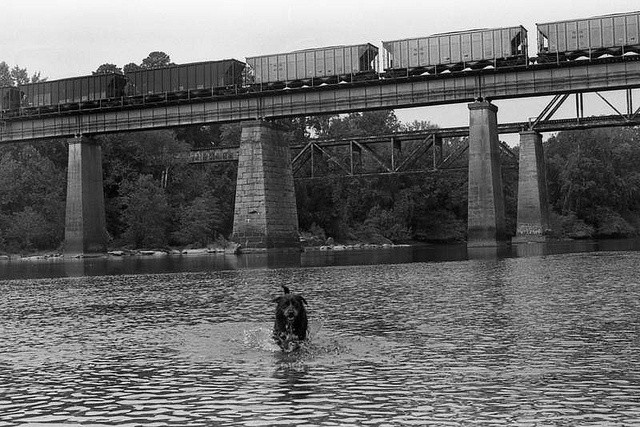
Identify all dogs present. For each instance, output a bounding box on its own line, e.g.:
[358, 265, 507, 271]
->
[268, 284, 308, 354]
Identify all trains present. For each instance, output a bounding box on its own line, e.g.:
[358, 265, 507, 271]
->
[23, 10, 640, 119]
